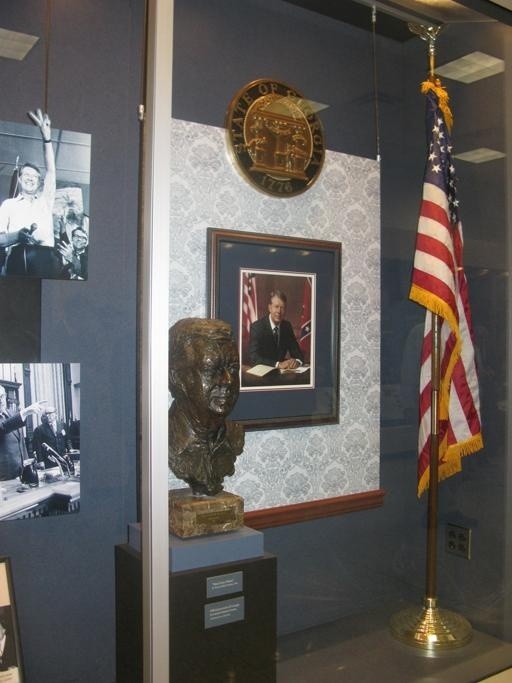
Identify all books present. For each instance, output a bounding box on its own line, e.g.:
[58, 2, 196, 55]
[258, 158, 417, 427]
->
[294, 367, 311, 373]
[246, 364, 300, 377]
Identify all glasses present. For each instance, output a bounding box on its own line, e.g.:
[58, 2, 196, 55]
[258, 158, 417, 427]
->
[46, 411, 56, 415]
[72, 234, 87, 240]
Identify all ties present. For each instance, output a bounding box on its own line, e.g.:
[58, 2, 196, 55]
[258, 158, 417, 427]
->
[271, 326, 279, 347]
[51, 423, 56, 435]
[2, 411, 10, 421]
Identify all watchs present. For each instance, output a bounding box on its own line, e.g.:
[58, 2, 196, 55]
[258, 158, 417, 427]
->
[44, 139, 51, 143]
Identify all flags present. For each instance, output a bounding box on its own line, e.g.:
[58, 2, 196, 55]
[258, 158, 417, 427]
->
[243, 273, 258, 350]
[297, 277, 312, 363]
[409, 78, 484, 498]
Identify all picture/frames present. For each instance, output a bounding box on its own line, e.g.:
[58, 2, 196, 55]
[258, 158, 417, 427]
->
[206, 227, 342, 432]
[0, 555, 25, 683]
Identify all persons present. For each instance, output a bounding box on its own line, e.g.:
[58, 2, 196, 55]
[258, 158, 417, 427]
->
[37, 406, 79, 469]
[168, 317, 245, 498]
[53, 195, 90, 281]
[32, 412, 47, 459]
[0, 385, 48, 481]
[248, 290, 304, 369]
[0, 108, 62, 279]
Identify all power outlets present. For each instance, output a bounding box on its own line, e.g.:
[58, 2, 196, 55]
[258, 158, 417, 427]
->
[444, 522, 472, 560]
